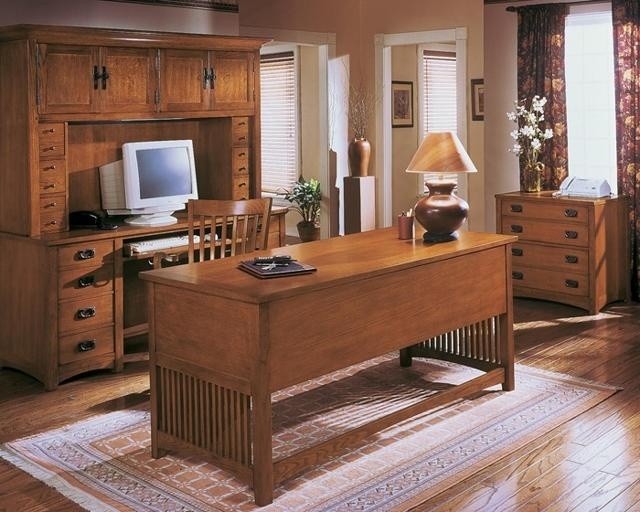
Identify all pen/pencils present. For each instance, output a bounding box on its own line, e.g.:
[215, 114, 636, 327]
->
[256, 263, 288, 267]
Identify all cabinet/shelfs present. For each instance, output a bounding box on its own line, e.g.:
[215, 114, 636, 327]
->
[157, 33, 263, 120]
[0, 24, 158, 120]
[494, 189, 632, 315]
[0, 122, 68, 230]
[197, 116, 252, 198]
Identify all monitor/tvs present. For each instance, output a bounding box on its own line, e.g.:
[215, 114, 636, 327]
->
[99, 139, 199, 227]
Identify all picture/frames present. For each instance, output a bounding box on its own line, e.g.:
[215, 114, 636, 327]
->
[392, 80, 415, 130]
[470, 78, 486, 120]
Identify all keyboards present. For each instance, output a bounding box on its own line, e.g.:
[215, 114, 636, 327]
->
[126, 234, 200, 252]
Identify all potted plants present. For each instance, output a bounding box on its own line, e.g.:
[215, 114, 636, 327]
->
[272, 173, 322, 243]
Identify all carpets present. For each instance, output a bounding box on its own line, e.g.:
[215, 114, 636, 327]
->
[0, 344, 624, 511]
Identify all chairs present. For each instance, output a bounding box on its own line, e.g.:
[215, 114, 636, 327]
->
[185, 197, 272, 264]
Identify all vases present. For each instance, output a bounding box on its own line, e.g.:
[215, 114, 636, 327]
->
[522, 159, 546, 193]
[348, 136, 372, 176]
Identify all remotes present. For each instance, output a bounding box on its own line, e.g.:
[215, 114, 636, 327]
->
[255, 255, 297, 264]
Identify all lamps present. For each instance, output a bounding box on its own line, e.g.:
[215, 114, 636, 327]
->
[406, 131, 480, 242]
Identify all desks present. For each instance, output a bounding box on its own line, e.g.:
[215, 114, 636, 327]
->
[1, 206, 289, 389]
[139, 225, 519, 505]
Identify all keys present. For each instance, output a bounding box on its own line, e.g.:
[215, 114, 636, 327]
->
[261, 264, 276, 270]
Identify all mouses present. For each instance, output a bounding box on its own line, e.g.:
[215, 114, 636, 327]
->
[205, 233, 218, 241]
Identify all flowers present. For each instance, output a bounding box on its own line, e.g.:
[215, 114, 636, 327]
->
[508, 94, 554, 163]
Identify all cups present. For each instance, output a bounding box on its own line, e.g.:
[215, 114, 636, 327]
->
[398, 215, 415, 240]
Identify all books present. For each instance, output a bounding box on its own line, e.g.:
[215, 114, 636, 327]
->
[238, 260, 316, 278]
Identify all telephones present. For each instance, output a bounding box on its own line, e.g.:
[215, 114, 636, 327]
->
[69, 210, 101, 227]
[559, 175, 611, 198]
